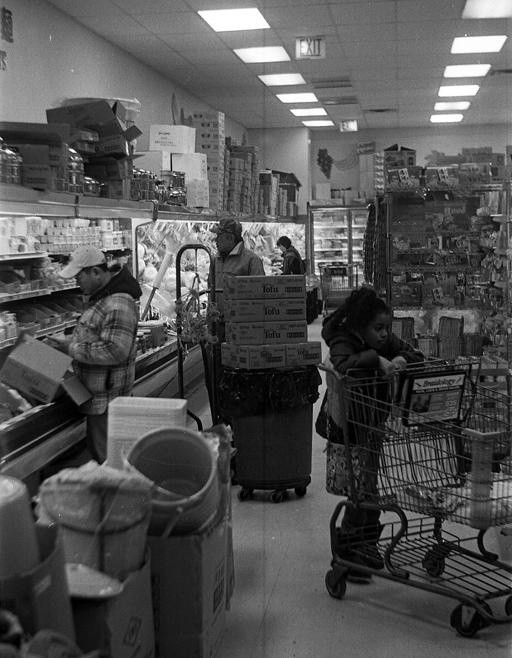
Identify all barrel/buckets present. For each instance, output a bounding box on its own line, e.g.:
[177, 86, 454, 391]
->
[120, 430, 222, 529]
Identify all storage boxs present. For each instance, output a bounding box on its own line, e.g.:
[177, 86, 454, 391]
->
[383, 144, 511, 191]
[66, 521, 237, 658]
[0, 95, 296, 215]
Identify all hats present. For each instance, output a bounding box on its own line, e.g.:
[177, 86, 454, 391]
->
[209, 219, 242, 234]
[59, 246, 107, 279]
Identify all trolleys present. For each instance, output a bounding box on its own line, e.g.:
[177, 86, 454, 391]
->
[315, 257, 365, 321]
[171, 233, 239, 491]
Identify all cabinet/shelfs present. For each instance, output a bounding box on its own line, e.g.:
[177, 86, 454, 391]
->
[0, 182, 512, 493]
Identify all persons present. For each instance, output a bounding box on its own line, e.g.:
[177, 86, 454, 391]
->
[203, 215, 266, 425]
[315, 285, 427, 569]
[274, 232, 308, 277]
[43, 242, 143, 464]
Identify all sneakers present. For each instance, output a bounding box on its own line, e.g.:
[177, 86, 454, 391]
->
[330, 546, 384, 585]
